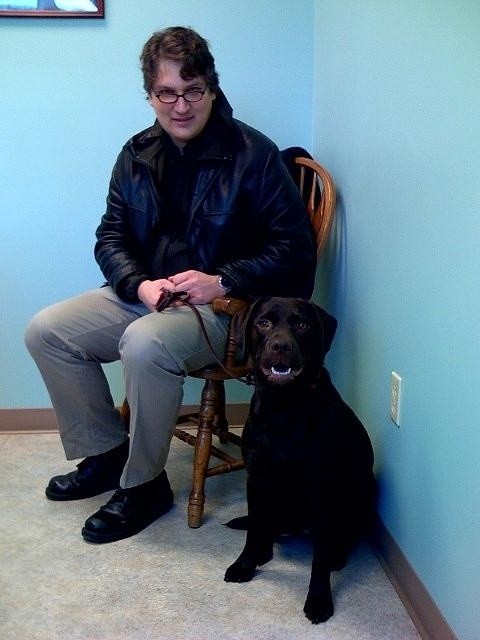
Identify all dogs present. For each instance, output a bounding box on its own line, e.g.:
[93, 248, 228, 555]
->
[223, 292, 380, 625]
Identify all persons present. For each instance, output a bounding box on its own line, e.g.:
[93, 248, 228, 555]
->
[23, 25, 315, 545]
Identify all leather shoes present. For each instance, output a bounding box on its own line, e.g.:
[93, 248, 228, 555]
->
[82, 469, 173, 543]
[46, 437, 130, 501]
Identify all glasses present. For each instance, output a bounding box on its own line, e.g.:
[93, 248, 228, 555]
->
[151, 83, 208, 104]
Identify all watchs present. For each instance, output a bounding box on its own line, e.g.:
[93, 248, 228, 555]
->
[219, 277, 233, 294]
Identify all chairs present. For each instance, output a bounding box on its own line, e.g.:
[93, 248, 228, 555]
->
[119, 158, 337, 531]
